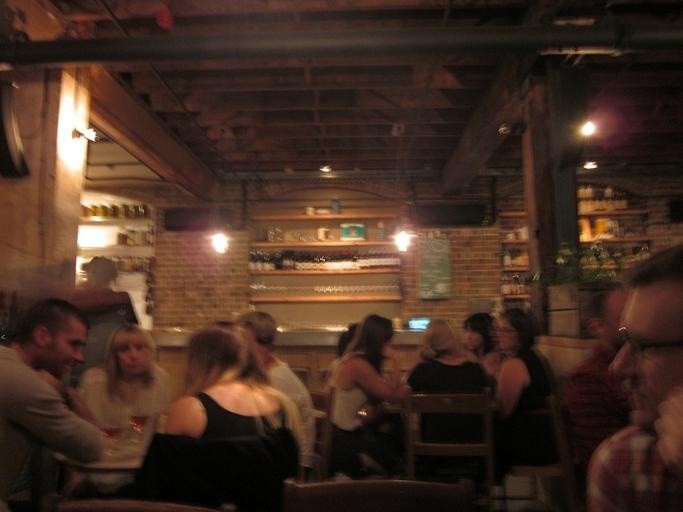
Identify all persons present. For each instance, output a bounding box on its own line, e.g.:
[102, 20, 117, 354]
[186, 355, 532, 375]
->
[1, 295, 174, 511]
[556, 281, 639, 512]
[581, 241, 683, 511]
[136, 310, 316, 511]
[74, 255, 139, 326]
[328, 313, 412, 479]
[407, 309, 555, 465]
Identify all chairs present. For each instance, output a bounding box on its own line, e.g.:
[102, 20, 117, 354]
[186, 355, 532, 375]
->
[57, 387, 495, 512]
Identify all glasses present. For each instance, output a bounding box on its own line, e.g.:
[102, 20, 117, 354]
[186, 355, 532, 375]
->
[614, 325, 682, 359]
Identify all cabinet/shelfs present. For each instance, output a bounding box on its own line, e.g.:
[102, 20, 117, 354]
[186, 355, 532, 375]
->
[247, 213, 402, 305]
[498, 210, 652, 304]
